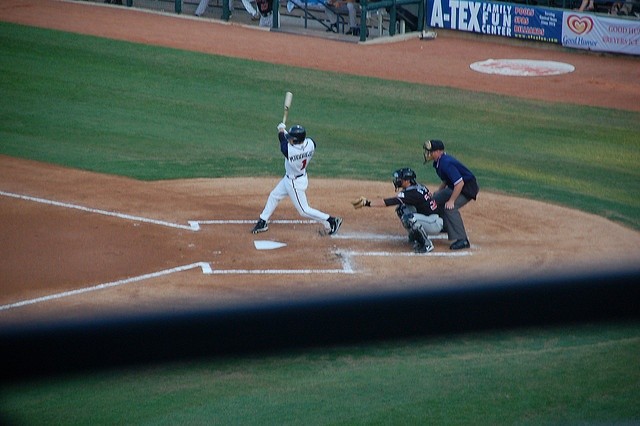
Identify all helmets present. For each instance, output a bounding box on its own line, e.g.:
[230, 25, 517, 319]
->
[285, 123, 305, 143]
[423, 139, 444, 164]
[393, 167, 416, 191]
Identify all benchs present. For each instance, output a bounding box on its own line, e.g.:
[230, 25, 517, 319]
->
[184, 1, 389, 37]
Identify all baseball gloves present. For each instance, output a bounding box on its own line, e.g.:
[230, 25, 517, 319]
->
[350, 196, 367, 210]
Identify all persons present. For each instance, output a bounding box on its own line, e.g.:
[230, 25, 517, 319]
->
[195, 0, 235, 19]
[351, 168, 443, 254]
[241, 0, 263, 19]
[256, 0, 280, 28]
[325, 0, 361, 35]
[422, 139, 479, 250]
[578, 0, 595, 13]
[250, 122, 343, 236]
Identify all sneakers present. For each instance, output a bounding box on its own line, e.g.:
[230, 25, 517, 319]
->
[450, 238, 470, 248]
[326, 23, 337, 30]
[328, 217, 343, 235]
[345, 27, 356, 33]
[251, 222, 268, 232]
[414, 240, 434, 253]
[408, 233, 416, 242]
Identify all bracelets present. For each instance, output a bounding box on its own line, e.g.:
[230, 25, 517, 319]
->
[365, 200, 372, 207]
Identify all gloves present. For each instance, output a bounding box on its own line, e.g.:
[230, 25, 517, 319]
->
[277, 122, 286, 128]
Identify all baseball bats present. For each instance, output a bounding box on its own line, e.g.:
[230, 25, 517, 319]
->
[282, 92, 293, 125]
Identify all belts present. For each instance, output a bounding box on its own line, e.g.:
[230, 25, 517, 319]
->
[287, 174, 303, 178]
[260, 11, 272, 16]
